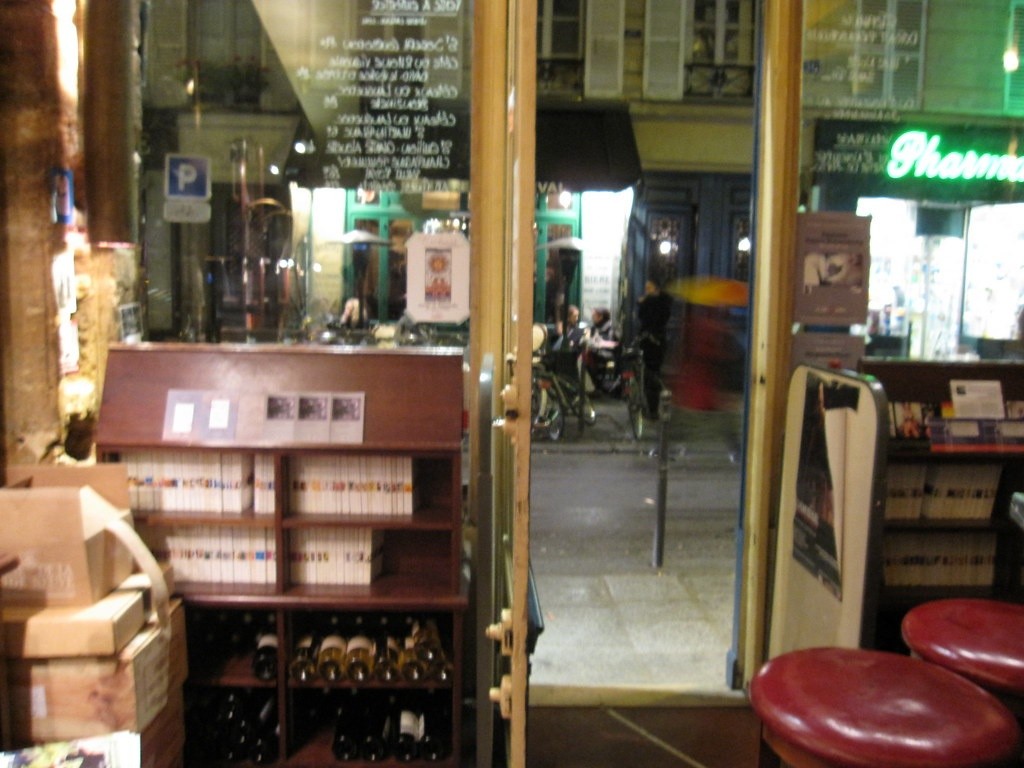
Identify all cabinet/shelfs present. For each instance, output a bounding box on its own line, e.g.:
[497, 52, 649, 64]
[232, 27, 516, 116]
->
[96, 341, 464, 768]
[766, 357, 1023, 666]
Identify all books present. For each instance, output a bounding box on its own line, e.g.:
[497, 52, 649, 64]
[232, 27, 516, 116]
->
[118, 447, 419, 585]
[881, 464, 1002, 586]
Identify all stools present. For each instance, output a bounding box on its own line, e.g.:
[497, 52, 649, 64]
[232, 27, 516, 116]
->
[901, 597, 1024, 710]
[749, 648, 1020, 768]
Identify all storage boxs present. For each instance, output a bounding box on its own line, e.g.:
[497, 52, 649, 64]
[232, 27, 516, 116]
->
[0, 462, 191, 768]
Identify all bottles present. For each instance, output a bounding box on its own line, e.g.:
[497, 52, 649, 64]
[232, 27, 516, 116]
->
[187, 689, 278, 764]
[250, 613, 278, 680]
[330, 688, 445, 761]
[289, 615, 445, 682]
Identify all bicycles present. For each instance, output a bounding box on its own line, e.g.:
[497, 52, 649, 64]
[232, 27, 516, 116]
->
[618, 333, 673, 444]
[323, 313, 599, 442]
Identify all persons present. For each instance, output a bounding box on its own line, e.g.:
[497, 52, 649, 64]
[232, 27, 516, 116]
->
[900, 402, 919, 438]
[556, 275, 673, 420]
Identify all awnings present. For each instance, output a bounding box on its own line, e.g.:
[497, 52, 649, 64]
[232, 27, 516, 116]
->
[280, 102, 641, 192]
[811, 117, 1024, 207]
